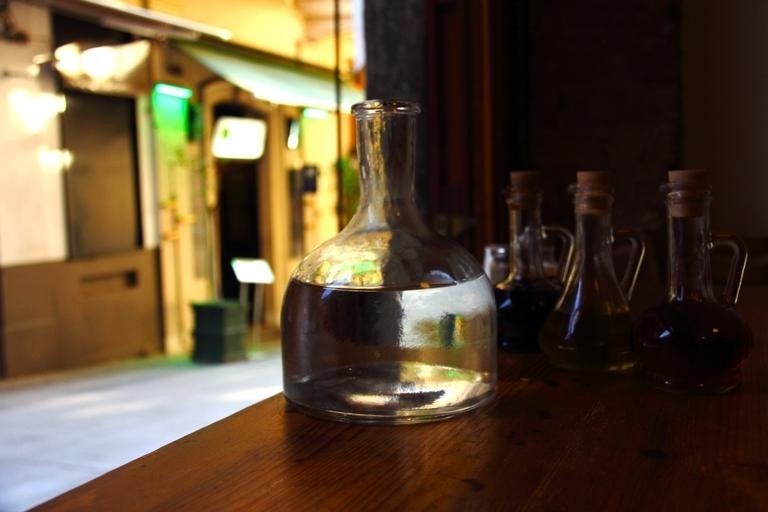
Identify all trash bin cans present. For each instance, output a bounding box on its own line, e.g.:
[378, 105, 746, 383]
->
[192, 300, 248, 365]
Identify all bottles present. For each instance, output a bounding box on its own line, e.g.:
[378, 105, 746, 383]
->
[539, 170, 646, 380]
[493, 171, 576, 357]
[277, 97, 511, 428]
[635, 167, 753, 396]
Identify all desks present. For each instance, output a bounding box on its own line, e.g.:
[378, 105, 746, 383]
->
[28, 278, 767, 511]
